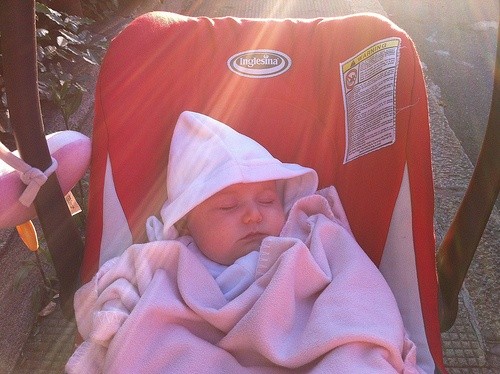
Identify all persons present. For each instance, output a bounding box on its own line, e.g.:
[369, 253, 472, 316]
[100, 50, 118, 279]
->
[66, 111, 419, 374]
